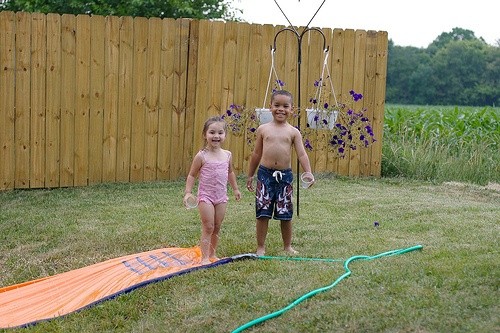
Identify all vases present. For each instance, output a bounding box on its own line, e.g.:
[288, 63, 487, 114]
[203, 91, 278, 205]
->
[306, 107, 338, 131]
[255, 108, 273, 125]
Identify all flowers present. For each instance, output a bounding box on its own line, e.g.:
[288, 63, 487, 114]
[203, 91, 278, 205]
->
[293, 76, 377, 160]
[219, 79, 293, 144]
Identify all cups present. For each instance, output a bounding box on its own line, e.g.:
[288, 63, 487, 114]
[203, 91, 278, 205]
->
[185, 196, 198, 209]
[301, 172, 314, 188]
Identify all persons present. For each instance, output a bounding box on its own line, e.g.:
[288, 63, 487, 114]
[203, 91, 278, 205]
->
[184, 115, 241, 266]
[246, 90, 316, 257]
